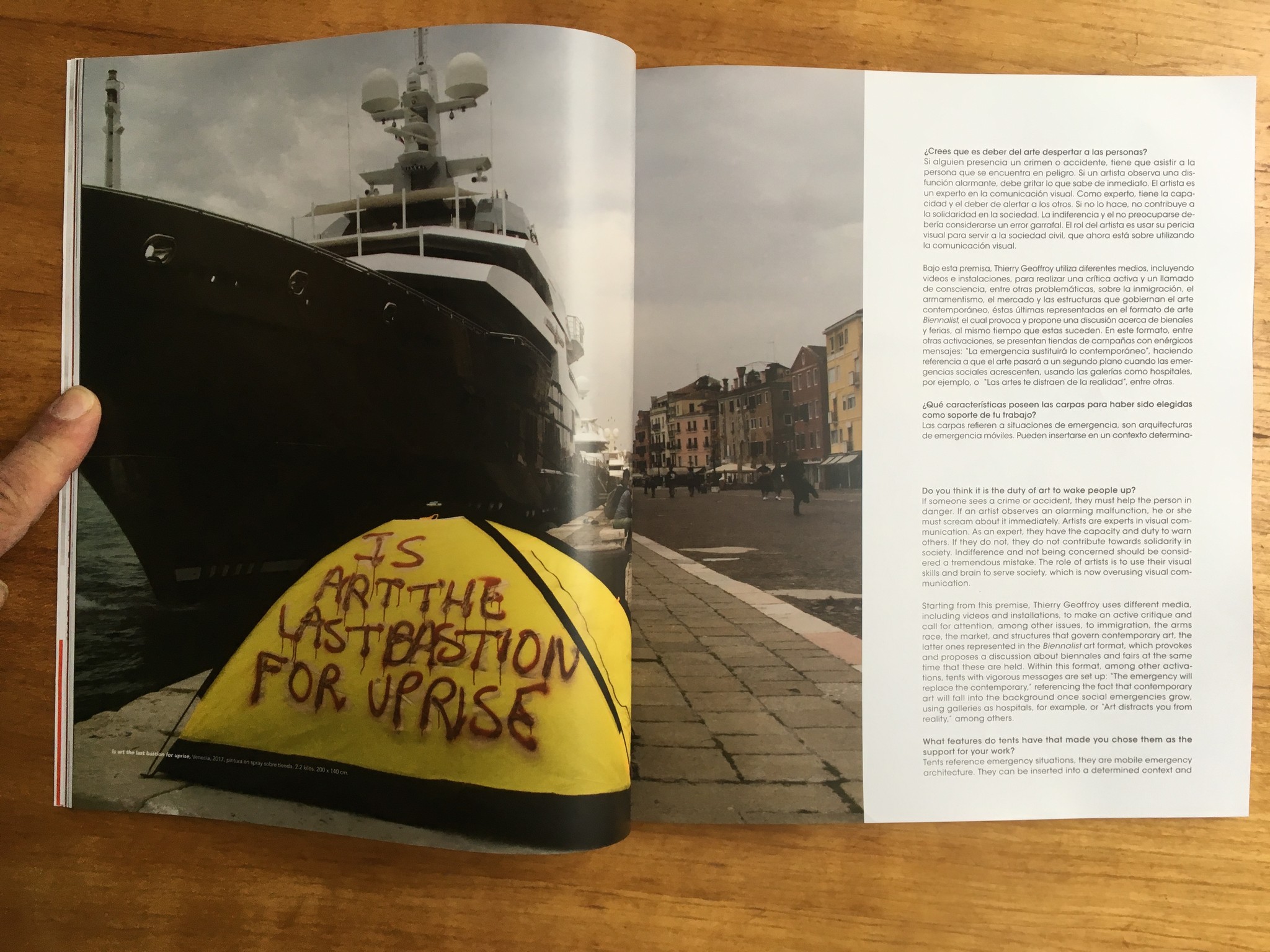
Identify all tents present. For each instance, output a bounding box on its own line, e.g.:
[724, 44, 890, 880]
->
[137, 499, 632, 849]
[705, 463, 757, 485]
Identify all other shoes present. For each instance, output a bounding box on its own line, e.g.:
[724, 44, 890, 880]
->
[794, 512, 801, 516]
[775, 496, 781, 500]
[763, 497, 768, 500]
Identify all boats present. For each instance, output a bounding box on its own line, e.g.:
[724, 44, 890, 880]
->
[78, 25, 632, 675]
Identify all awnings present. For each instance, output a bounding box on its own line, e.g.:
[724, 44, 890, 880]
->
[756, 461, 821, 471]
[647, 467, 702, 477]
[820, 455, 858, 465]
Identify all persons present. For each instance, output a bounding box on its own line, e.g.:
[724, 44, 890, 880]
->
[728, 476, 732, 483]
[781, 451, 819, 516]
[665, 466, 676, 498]
[757, 460, 782, 500]
[608, 468, 633, 554]
[686, 467, 696, 497]
[0, 386, 101, 611]
[644, 478, 657, 498]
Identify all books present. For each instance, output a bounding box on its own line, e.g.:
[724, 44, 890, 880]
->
[54, 25, 1254, 851]
